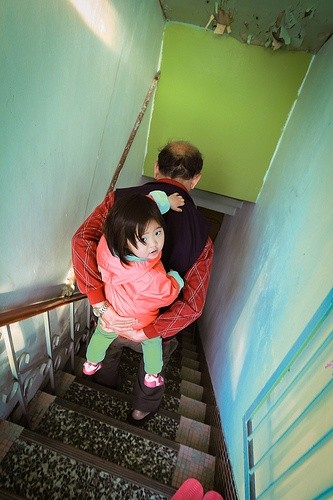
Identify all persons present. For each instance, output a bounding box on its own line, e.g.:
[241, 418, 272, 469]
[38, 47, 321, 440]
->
[72, 140, 214, 424]
[83, 189, 185, 388]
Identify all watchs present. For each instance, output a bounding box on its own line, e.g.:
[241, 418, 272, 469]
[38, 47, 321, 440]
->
[91, 301, 110, 317]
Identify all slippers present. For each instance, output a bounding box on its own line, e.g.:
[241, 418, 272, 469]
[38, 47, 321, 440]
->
[170, 478, 224, 500]
[127, 409, 158, 425]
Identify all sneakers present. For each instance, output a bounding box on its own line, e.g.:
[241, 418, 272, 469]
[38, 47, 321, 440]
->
[82, 361, 103, 375]
[144, 374, 164, 388]
